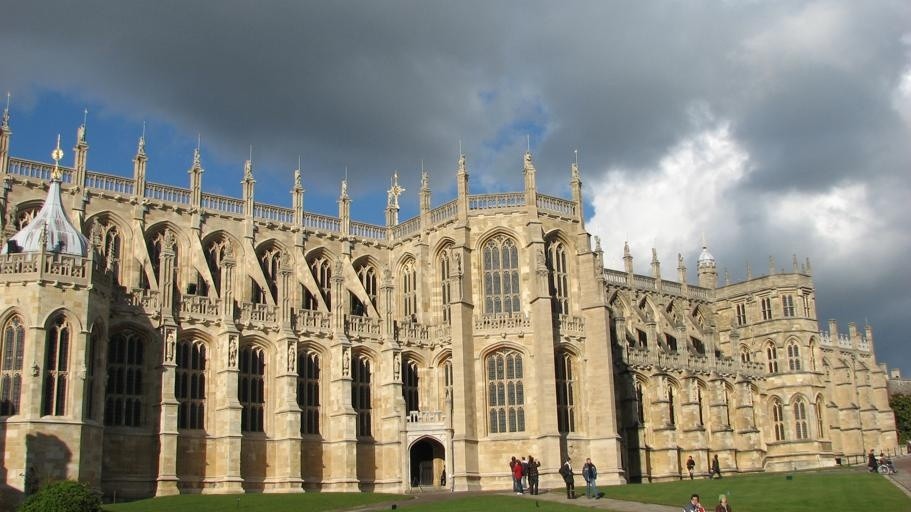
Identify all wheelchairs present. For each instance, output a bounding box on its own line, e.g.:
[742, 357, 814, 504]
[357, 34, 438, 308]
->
[876, 457, 896, 475]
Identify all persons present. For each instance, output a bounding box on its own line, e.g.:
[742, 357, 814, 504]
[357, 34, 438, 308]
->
[510, 455, 540, 495]
[684, 494, 705, 512]
[582, 458, 600, 499]
[710, 455, 721, 478]
[868, 449, 896, 475]
[687, 456, 695, 480]
[441, 465, 446, 486]
[563, 457, 577, 499]
[715, 494, 732, 512]
